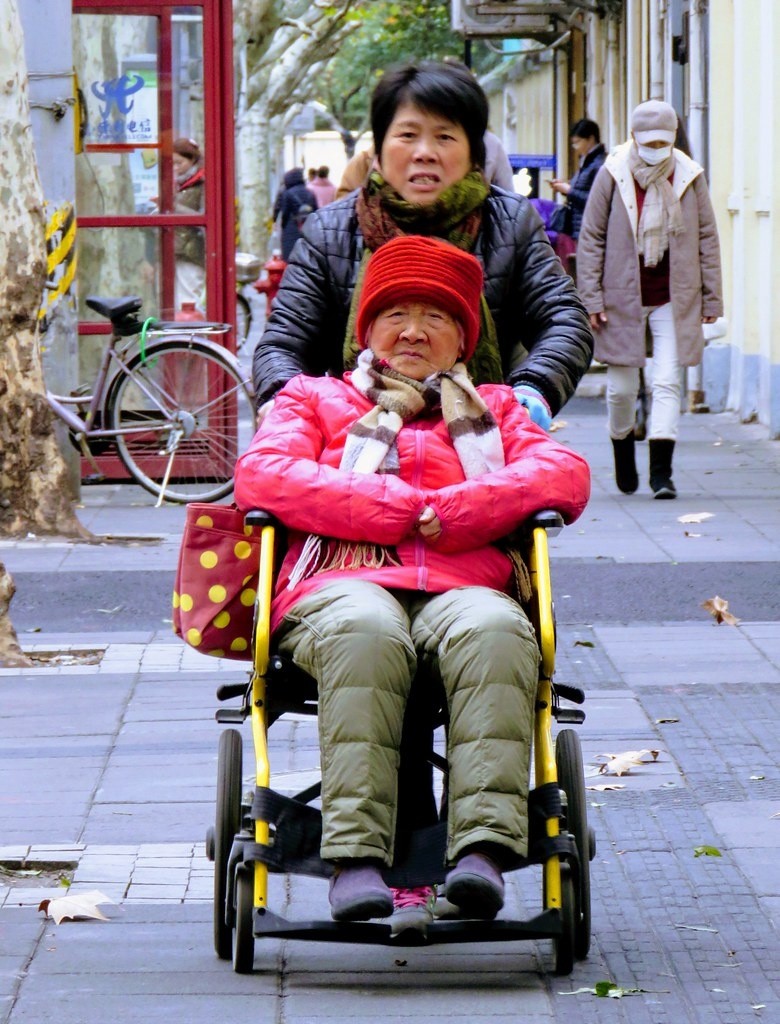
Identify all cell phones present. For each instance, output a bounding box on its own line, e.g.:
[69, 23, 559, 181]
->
[544, 179, 553, 185]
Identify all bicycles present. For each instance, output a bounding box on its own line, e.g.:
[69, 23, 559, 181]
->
[174, 262, 254, 352]
[43, 297, 262, 507]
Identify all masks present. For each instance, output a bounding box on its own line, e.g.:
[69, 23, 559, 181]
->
[635, 138, 672, 166]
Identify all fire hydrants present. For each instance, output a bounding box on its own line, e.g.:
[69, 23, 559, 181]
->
[253, 249, 286, 323]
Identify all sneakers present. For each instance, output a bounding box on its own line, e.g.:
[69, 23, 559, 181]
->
[379, 881, 458, 934]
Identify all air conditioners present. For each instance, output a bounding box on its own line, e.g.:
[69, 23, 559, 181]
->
[449, 0, 549, 35]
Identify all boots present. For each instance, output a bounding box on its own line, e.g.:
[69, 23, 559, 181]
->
[610, 428, 638, 494]
[648, 438, 678, 499]
[687, 390, 709, 412]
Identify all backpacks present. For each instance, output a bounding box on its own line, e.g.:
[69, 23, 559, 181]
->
[287, 187, 313, 233]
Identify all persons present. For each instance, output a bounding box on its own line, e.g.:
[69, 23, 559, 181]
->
[148, 139, 207, 313]
[549, 112, 610, 374]
[575, 97, 724, 496]
[251, 52, 596, 427]
[231, 233, 593, 919]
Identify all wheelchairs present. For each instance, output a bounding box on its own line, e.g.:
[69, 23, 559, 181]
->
[205, 510, 599, 977]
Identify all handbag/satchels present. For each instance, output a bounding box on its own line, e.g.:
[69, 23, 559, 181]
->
[550, 201, 573, 236]
[173, 502, 264, 662]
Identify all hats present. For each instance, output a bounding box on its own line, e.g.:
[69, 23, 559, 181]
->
[174, 137, 201, 164]
[631, 99, 678, 144]
[356, 235, 484, 364]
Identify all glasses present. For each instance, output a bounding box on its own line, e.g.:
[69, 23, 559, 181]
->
[572, 139, 585, 149]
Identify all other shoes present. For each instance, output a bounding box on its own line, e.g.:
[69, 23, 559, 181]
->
[443, 851, 506, 920]
[635, 408, 647, 440]
[328, 864, 395, 923]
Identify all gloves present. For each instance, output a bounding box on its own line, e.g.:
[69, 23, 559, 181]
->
[512, 384, 553, 433]
